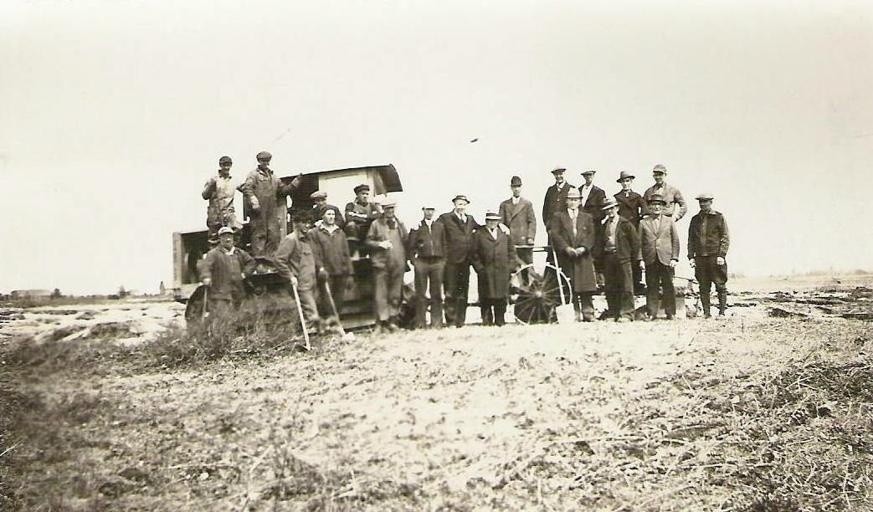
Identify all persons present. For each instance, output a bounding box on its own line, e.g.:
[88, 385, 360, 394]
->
[688, 193, 729, 318]
[543, 164, 687, 322]
[200, 153, 537, 336]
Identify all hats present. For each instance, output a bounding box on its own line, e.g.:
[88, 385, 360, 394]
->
[485, 212, 502, 219]
[580, 171, 597, 174]
[452, 195, 470, 204]
[566, 188, 583, 199]
[354, 184, 369, 193]
[310, 191, 327, 200]
[380, 201, 397, 209]
[219, 156, 232, 164]
[695, 194, 713, 200]
[616, 171, 634, 182]
[217, 227, 236, 235]
[511, 176, 521, 186]
[653, 164, 666, 173]
[599, 197, 618, 210]
[257, 152, 272, 159]
[648, 194, 666, 206]
[551, 167, 566, 172]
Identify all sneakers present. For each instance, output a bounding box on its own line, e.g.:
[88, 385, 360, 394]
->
[701, 314, 727, 319]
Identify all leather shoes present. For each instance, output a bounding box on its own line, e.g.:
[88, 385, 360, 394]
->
[575, 313, 676, 321]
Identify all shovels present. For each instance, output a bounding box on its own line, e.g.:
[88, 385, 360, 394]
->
[546, 229, 576, 324]
